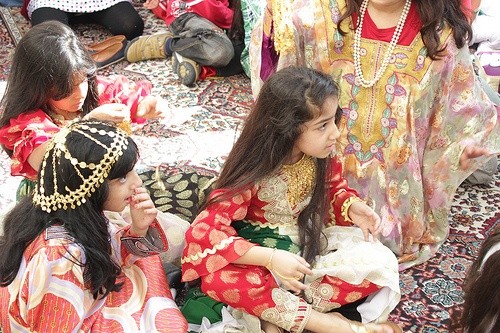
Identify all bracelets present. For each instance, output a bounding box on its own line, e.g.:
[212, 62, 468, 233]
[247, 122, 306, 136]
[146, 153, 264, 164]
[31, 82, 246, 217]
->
[266, 247, 277, 270]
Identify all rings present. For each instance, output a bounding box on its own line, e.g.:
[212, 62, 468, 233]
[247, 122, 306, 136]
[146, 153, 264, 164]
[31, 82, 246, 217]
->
[297, 273, 302, 280]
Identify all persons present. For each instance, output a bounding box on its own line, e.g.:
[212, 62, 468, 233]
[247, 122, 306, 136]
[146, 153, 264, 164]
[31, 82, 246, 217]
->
[180, 66, 400, 332]
[27, 0, 145, 40]
[249, 0, 500, 271]
[0, 21, 192, 262]
[1, 118, 188, 333]
[123, 1, 244, 85]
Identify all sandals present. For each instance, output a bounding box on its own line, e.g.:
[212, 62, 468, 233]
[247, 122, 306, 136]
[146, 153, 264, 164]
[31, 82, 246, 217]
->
[330, 312, 403, 333]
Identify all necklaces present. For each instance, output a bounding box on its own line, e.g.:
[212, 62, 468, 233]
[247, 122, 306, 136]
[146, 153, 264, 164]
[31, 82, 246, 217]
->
[354, 0, 412, 87]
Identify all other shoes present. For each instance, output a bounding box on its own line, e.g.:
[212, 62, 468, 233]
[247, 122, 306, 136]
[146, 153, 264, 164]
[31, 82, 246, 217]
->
[169, 52, 199, 86]
[125, 31, 173, 64]
[85, 34, 127, 67]
